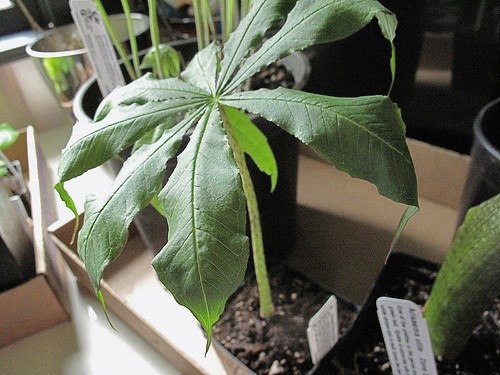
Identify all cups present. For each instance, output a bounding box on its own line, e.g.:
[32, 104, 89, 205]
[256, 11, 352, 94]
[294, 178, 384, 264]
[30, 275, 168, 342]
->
[452, 98, 500, 240]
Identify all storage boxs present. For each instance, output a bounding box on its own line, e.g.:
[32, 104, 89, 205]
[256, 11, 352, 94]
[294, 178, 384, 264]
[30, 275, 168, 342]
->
[0, 124, 72, 352]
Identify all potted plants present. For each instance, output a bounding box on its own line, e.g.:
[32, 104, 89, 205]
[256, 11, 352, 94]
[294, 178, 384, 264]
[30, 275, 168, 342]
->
[10, 0, 500, 375]
